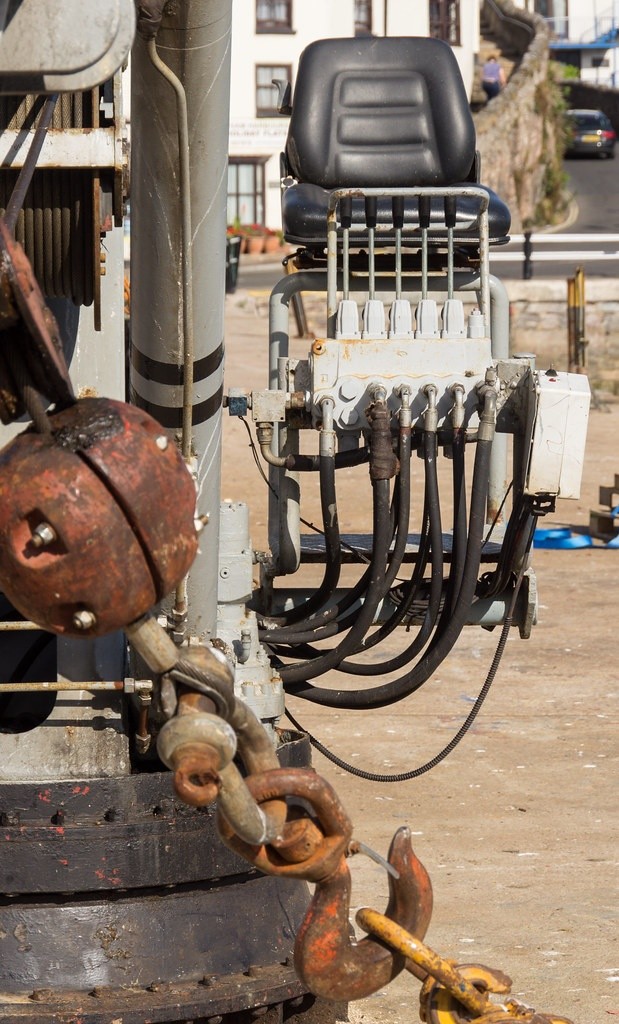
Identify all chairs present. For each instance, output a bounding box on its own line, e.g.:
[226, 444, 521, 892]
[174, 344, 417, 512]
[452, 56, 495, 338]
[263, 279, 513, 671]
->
[280, 36, 511, 245]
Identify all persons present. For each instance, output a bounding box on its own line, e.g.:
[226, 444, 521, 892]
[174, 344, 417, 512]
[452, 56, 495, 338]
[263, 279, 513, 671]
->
[482, 57, 505, 100]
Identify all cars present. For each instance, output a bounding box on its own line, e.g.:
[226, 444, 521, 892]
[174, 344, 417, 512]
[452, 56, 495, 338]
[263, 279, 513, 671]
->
[562, 109, 616, 159]
